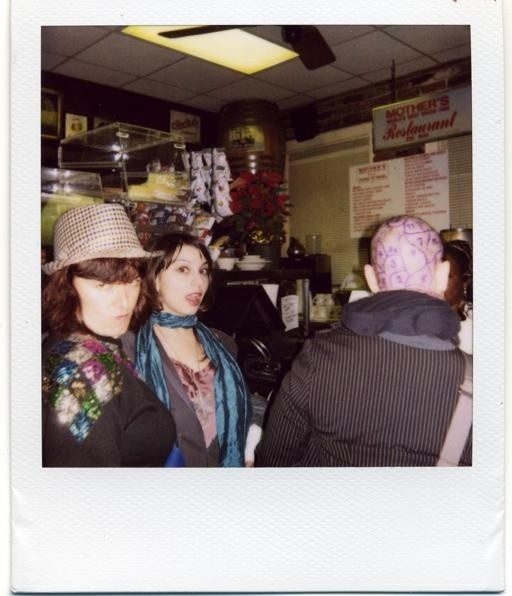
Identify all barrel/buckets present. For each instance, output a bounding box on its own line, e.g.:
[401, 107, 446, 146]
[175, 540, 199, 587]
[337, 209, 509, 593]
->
[214, 98, 288, 181]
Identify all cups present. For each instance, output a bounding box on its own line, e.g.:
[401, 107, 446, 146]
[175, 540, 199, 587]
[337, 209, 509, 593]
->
[305, 233, 322, 255]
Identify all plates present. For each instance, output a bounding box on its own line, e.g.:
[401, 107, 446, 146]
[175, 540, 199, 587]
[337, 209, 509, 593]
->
[236, 260, 274, 271]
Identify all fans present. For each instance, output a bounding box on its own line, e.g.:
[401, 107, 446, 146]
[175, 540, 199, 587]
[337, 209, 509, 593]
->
[158, 27, 338, 71]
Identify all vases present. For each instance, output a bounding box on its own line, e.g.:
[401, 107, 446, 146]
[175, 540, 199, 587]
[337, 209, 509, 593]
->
[248, 241, 281, 267]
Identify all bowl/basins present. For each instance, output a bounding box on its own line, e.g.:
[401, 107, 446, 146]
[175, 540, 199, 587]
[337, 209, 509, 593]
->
[215, 258, 235, 271]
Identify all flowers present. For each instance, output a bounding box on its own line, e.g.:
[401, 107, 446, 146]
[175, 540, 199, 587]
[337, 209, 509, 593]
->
[225, 165, 294, 246]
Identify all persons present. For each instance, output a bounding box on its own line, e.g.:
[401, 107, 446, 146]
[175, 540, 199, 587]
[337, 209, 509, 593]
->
[242, 128, 256, 146]
[247, 215, 473, 467]
[118, 233, 252, 467]
[231, 131, 246, 147]
[43, 202, 176, 466]
[438, 239, 472, 354]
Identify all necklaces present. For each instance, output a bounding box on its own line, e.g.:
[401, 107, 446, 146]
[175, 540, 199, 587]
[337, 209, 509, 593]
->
[155, 324, 202, 372]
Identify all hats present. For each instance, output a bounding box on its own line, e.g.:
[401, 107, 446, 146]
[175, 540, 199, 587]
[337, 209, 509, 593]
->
[42, 203, 166, 276]
